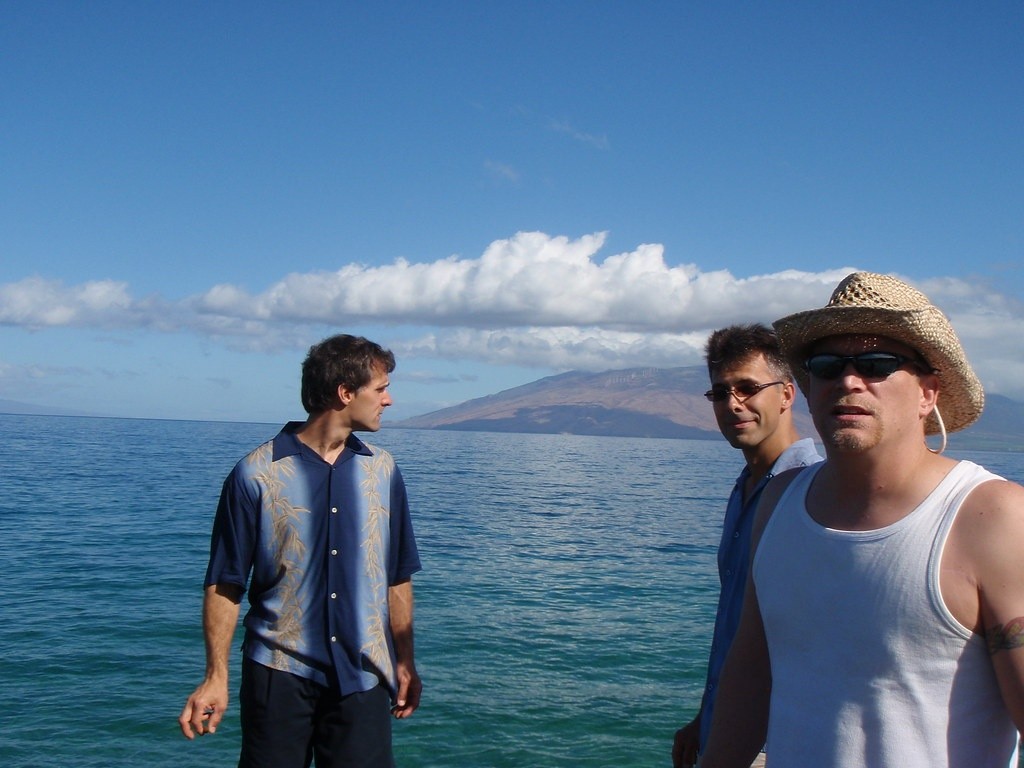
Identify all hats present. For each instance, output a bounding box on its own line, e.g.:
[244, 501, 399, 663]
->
[771, 272, 986, 436]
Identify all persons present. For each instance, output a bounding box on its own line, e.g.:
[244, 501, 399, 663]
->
[178, 333, 422, 768]
[673, 325, 824, 768]
[699, 273, 1024, 768]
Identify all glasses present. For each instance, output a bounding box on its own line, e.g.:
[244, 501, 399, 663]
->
[704, 381, 785, 401]
[797, 349, 929, 379]
